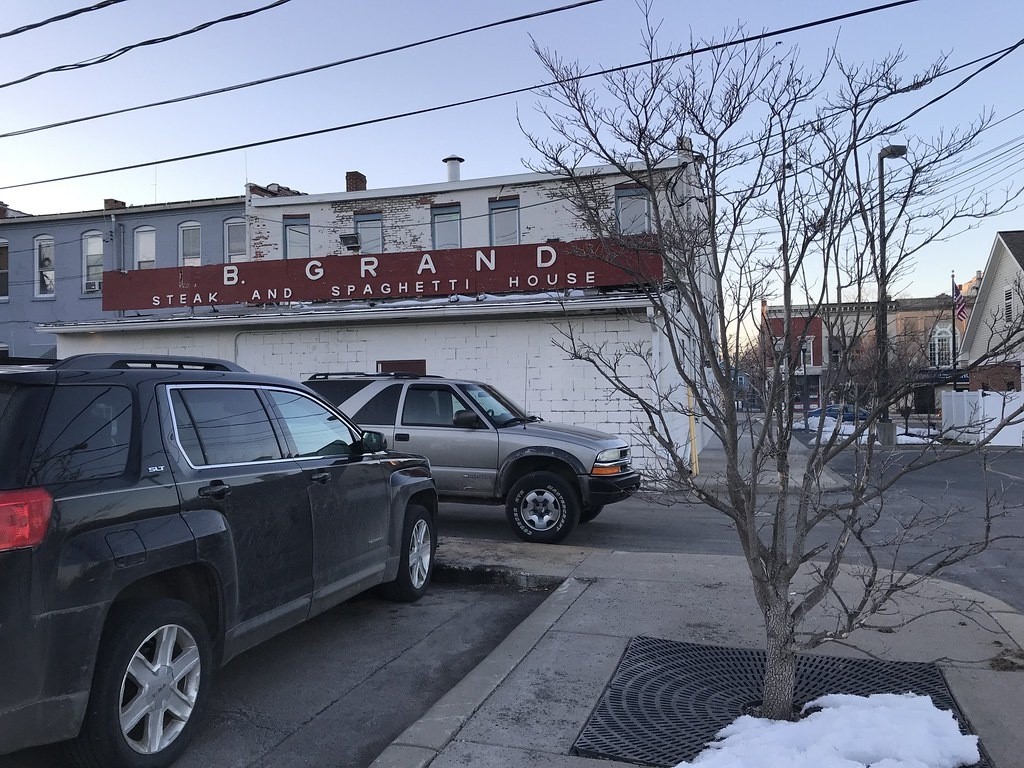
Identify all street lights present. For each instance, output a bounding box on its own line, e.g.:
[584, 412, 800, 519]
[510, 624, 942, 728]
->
[876, 141, 908, 447]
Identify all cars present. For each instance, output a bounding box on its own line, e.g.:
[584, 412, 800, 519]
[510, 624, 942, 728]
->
[804, 404, 894, 424]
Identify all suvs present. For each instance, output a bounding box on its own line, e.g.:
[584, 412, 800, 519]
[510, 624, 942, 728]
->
[299, 369, 643, 545]
[2, 353, 446, 768]
